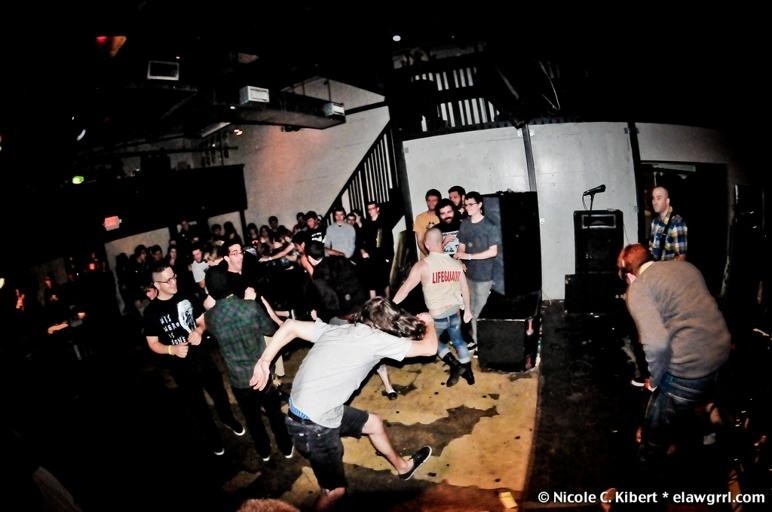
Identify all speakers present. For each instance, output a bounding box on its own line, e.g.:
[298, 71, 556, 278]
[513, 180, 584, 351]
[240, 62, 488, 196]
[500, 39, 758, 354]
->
[574, 209, 624, 273]
[483, 192, 543, 297]
[565, 274, 633, 337]
[476, 293, 540, 371]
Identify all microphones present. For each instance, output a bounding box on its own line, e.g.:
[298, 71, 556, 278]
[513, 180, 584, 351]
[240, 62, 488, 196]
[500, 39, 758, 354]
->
[583, 185, 606, 197]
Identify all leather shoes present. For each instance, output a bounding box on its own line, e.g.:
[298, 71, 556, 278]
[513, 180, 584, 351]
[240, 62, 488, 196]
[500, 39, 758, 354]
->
[382, 389, 397, 400]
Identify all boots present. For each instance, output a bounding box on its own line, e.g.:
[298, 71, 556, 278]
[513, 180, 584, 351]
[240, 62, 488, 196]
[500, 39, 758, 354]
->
[441, 352, 466, 387]
[461, 361, 475, 385]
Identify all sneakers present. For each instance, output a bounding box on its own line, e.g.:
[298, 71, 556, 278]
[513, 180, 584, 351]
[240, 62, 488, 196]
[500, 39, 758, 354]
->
[263, 447, 272, 462]
[399, 445, 433, 481]
[285, 445, 295, 459]
[208, 439, 226, 456]
[218, 416, 246, 438]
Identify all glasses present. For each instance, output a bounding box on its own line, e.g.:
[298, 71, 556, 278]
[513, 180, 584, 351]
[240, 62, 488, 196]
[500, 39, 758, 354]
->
[228, 250, 246, 256]
[154, 273, 177, 284]
[464, 202, 480, 206]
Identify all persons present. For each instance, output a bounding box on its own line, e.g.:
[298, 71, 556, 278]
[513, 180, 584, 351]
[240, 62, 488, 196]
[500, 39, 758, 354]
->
[645, 186, 691, 261]
[617, 241, 734, 454]
[9, 183, 500, 512]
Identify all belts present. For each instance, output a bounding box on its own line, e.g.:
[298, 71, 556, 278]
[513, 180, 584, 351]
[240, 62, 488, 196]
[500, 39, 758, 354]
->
[434, 313, 458, 323]
[287, 408, 315, 425]
